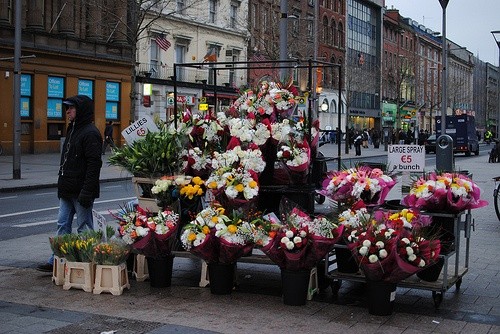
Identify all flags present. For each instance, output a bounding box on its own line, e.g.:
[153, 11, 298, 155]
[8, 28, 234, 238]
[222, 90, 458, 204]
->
[155, 34, 171, 51]
[252, 51, 265, 64]
[204, 46, 217, 62]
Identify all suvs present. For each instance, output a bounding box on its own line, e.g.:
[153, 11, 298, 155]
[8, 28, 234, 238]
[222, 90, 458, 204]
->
[435, 114, 481, 158]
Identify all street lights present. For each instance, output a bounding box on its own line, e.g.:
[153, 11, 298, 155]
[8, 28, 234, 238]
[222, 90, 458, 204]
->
[491, 31, 500, 164]
[439, 0, 450, 141]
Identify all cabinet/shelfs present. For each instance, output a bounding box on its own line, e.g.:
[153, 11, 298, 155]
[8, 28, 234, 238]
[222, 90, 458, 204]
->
[170, 61, 340, 265]
[324, 207, 475, 306]
[49, 83, 487, 282]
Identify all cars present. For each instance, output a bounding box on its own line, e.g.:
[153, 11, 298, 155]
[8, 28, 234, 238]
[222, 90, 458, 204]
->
[425, 133, 436, 155]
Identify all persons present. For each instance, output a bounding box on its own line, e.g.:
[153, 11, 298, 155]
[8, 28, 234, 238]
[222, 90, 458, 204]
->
[102, 121, 118, 155]
[37, 95, 103, 272]
[349, 127, 429, 155]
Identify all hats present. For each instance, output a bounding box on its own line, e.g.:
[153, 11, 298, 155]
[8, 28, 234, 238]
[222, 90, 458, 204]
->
[62, 100, 76, 106]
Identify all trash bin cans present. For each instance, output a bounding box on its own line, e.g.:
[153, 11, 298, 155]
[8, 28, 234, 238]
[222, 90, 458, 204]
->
[436, 134, 453, 170]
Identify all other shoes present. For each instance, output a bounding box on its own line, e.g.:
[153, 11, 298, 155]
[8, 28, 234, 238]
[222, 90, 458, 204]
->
[35, 263, 53, 272]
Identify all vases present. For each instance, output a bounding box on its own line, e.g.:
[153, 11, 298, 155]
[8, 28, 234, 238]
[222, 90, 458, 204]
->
[146, 257, 174, 286]
[94, 261, 128, 295]
[368, 282, 397, 316]
[62, 261, 95, 292]
[281, 268, 308, 305]
[52, 256, 67, 286]
[207, 262, 237, 295]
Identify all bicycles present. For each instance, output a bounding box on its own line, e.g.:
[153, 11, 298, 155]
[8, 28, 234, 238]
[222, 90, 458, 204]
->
[492, 176, 500, 222]
